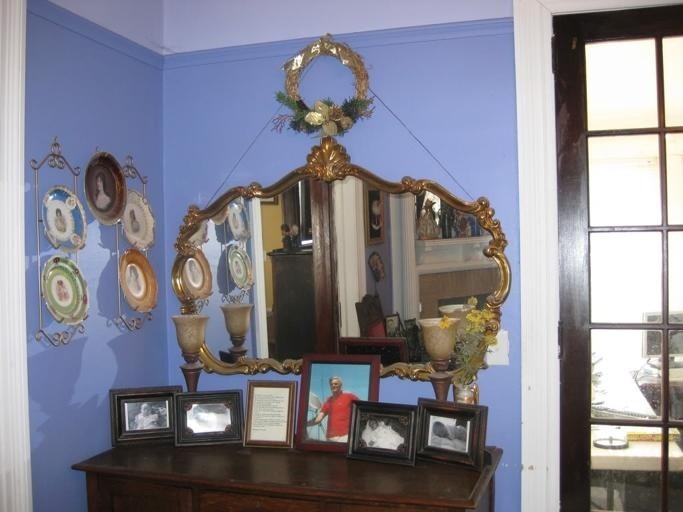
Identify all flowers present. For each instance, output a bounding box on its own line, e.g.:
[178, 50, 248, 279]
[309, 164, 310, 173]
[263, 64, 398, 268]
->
[439, 306, 497, 387]
[270, 89, 376, 138]
[437, 296, 478, 330]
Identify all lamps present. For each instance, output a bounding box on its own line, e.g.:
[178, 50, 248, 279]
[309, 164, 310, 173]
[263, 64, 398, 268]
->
[172, 314, 210, 393]
[438, 302, 476, 354]
[416, 315, 463, 402]
[219, 303, 254, 363]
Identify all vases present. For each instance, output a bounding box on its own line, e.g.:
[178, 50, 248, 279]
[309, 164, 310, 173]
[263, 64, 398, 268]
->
[416, 198, 440, 240]
[452, 383, 479, 405]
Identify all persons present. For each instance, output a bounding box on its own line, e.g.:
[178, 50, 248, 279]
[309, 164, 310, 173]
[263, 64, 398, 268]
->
[188, 260, 201, 284]
[231, 210, 242, 234]
[127, 207, 142, 232]
[290, 225, 302, 249]
[52, 279, 74, 303]
[50, 205, 71, 232]
[233, 259, 242, 276]
[305, 376, 362, 443]
[279, 223, 292, 250]
[124, 264, 144, 296]
[90, 172, 114, 210]
[430, 419, 468, 442]
[132, 401, 160, 429]
[367, 413, 405, 450]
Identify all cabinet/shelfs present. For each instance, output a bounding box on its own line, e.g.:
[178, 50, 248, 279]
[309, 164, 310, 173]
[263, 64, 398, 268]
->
[264, 240, 316, 363]
[413, 233, 502, 276]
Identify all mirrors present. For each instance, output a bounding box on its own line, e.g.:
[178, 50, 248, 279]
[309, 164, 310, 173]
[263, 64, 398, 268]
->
[171, 135, 510, 382]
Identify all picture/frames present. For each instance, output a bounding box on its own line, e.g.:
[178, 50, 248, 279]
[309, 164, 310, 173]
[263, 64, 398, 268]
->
[345, 398, 418, 469]
[108, 385, 183, 449]
[383, 312, 400, 337]
[363, 186, 386, 247]
[416, 398, 488, 472]
[296, 354, 380, 454]
[174, 389, 244, 448]
[337, 338, 406, 368]
[439, 200, 480, 239]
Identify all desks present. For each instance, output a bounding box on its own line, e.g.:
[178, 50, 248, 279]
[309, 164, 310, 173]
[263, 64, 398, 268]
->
[70, 445, 502, 512]
[591, 367, 683, 511]
[635, 364, 683, 419]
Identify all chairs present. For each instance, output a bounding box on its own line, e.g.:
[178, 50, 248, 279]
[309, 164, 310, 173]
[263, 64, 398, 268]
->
[354, 287, 384, 337]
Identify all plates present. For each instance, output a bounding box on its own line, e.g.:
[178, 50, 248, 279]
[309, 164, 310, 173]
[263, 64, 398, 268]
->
[228, 201, 247, 240]
[40, 257, 90, 326]
[42, 185, 87, 251]
[182, 250, 213, 298]
[82, 152, 128, 225]
[227, 244, 252, 290]
[122, 189, 156, 250]
[118, 247, 158, 313]
